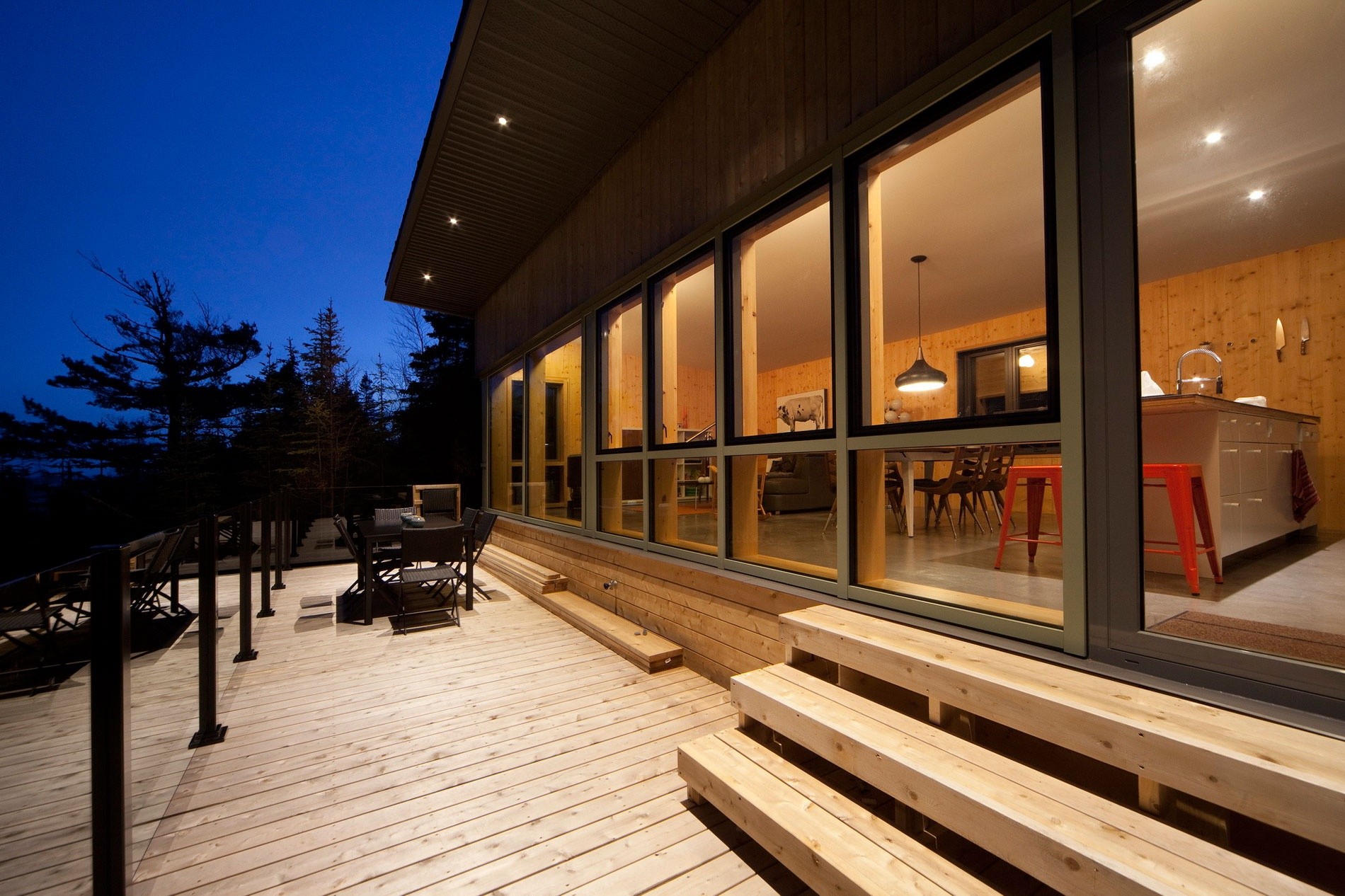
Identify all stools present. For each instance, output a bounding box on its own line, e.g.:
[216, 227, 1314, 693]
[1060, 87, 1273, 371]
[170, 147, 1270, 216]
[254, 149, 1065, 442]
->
[1142, 464, 1225, 595]
[995, 464, 1063, 570]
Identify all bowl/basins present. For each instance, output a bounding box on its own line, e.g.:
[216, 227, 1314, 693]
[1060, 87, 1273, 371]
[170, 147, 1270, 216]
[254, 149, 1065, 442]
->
[400, 512, 425, 528]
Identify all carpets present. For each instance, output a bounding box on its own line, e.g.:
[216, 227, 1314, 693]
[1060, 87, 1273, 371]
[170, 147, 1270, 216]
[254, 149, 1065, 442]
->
[623, 502, 715, 516]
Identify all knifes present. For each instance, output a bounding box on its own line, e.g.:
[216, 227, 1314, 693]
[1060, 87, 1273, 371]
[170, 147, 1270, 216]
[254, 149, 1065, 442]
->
[1300, 316, 1310, 355]
[1275, 317, 1286, 362]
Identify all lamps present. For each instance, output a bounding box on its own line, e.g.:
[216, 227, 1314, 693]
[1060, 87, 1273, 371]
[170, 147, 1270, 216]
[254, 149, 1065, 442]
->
[895, 255, 947, 390]
[884, 399, 910, 423]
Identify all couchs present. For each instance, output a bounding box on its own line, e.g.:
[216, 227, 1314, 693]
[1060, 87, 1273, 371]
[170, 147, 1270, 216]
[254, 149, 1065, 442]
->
[762, 452, 837, 512]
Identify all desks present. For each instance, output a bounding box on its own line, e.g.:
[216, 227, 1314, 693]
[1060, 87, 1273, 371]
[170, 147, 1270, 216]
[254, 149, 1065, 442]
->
[885, 445, 991, 538]
[357, 514, 474, 626]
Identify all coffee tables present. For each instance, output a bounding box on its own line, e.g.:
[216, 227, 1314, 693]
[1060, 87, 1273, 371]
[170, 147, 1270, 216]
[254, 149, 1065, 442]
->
[677, 480, 714, 510]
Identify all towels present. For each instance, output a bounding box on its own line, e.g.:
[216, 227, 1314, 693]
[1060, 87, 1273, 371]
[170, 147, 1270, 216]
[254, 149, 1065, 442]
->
[1291, 449, 1320, 524]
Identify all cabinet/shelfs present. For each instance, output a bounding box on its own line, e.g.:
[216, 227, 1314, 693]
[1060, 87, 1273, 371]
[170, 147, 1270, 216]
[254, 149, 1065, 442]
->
[1142, 410, 1319, 579]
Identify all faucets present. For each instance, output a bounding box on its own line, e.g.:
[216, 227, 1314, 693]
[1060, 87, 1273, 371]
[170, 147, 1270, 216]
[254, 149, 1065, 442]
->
[1176, 348, 1222, 394]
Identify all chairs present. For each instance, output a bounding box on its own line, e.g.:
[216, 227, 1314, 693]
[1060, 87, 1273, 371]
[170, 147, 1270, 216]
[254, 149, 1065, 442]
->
[333, 493, 396, 610]
[418, 507, 480, 594]
[958, 444, 1018, 532]
[0, 512, 259, 698]
[708, 454, 771, 520]
[823, 464, 904, 534]
[394, 524, 464, 636]
[431, 510, 499, 608]
[904, 446, 985, 537]
